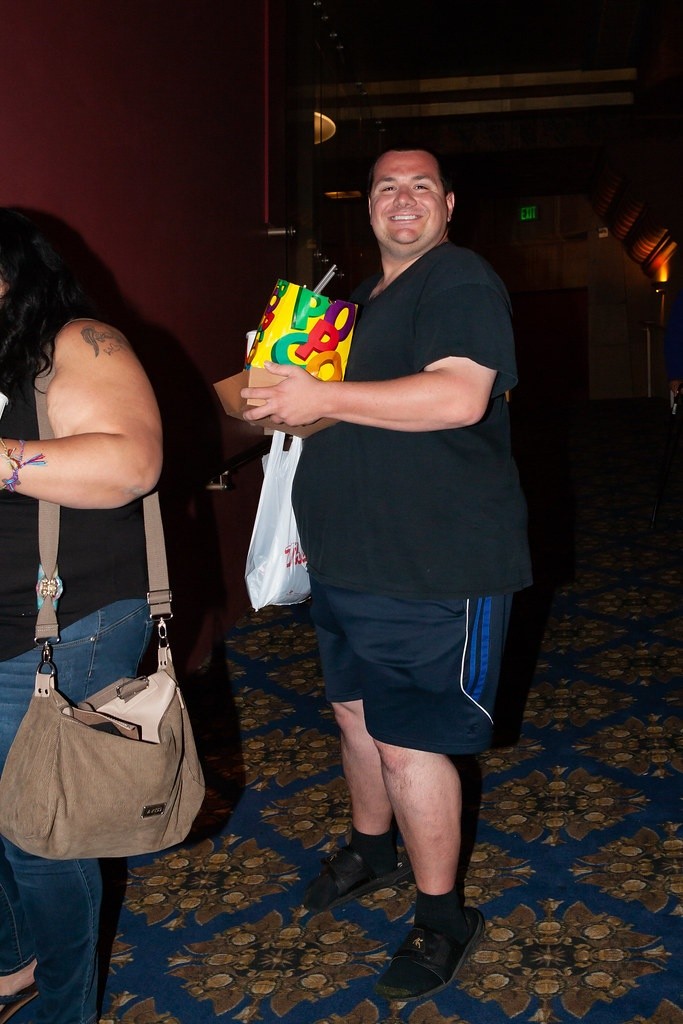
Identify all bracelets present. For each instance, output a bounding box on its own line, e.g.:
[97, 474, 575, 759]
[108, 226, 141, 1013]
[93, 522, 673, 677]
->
[0, 437, 48, 492]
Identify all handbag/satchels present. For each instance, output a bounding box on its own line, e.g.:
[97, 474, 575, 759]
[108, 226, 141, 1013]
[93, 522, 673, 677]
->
[0, 646, 207, 862]
[244, 429, 315, 613]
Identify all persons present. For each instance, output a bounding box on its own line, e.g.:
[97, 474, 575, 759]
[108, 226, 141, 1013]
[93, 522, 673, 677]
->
[240, 134, 534, 1005]
[0, 205, 164, 1024]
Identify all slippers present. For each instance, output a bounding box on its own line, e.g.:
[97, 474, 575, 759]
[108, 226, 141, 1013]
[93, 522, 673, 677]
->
[374, 906, 484, 1002]
[0, 980, 41, 1024]
[302, 846, 417, 913]
